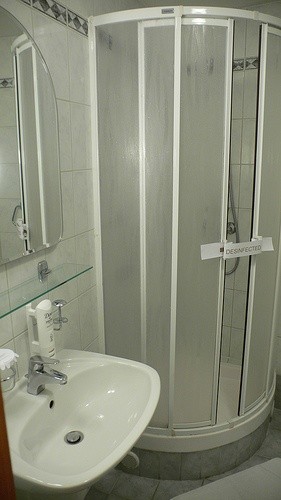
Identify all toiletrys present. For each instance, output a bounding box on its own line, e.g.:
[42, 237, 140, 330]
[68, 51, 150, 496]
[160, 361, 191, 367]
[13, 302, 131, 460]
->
[24, 300, 59, 367]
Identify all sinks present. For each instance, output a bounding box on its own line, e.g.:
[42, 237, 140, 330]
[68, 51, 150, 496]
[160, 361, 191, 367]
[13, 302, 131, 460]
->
[3, 348, 162, 500]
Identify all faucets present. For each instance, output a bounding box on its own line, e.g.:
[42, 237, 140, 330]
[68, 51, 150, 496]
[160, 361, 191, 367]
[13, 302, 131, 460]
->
[24, 356, 67, 396]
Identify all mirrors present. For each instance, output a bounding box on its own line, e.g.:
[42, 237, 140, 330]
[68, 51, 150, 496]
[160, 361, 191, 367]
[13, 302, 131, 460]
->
[0, 3, 63, 269]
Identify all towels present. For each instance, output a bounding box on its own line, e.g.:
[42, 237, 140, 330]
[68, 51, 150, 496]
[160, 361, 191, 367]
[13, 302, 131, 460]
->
[167, 455, 281, 499]
